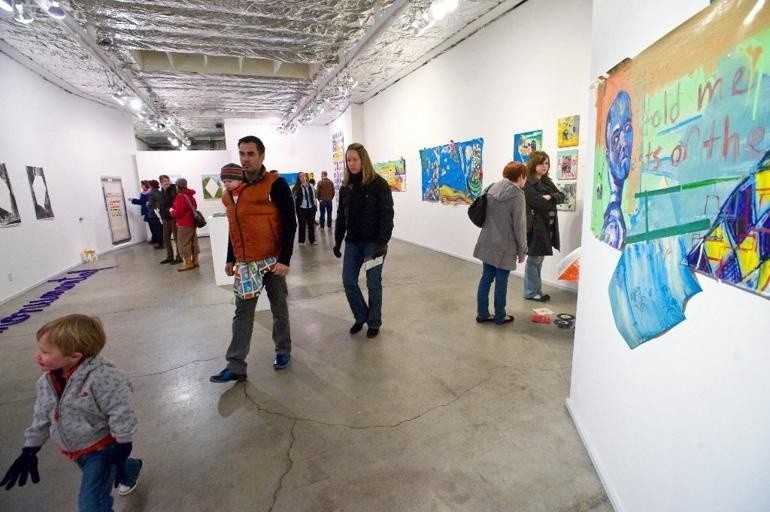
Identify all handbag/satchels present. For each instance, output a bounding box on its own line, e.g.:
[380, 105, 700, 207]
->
[468, 195, 486, 227]
[193, 211, 206, 227]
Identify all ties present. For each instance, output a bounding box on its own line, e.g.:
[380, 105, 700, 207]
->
[303, 185, 310, 207]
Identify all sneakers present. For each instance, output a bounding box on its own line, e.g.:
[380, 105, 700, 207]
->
[119, 458, 143, 494]
[161, 259, 172, 263]
[170, 260, 182, 265]
[368, 327, 378, 337]
[210, 369, 246, 383]
[273, 352, 289, 369]
[350, 321, 364, 333]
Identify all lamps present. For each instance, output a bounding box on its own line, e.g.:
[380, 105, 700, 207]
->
[397, 0, 459, 37]
[112, 83, 188, 152]
[294, 65, 357, 127]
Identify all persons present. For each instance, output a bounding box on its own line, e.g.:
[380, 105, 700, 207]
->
[129, 175, 200, 271]
[333, 143, 395, 339]
[293, 172, 318, 246]
[220, 163, 244, 190]
[521, 150, 567, 302]
[599, 88, 637, 249]
[471, 160, 528, 326]
[316, 171, 335, 229]
[0, 313, 144, 512]
[210, 136, 297, 384]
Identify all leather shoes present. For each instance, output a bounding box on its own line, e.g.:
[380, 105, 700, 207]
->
[503, 314, 513, 321]
[527, 293, 550, 302]
[477, 315, 494, 323]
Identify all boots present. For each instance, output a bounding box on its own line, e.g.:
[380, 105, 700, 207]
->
[177, 257, 198, 271]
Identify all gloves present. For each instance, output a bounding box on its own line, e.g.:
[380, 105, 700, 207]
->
[1, 446, 40, 490]
[102, 442, 131, 486]
[334, 244, 341, 258]
[374, 243, 385, 258]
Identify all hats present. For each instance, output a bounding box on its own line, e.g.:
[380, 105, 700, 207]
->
[149, 180, 159, 188]
[177, 179, 186, 188]
[221, 163, 244, 180]
